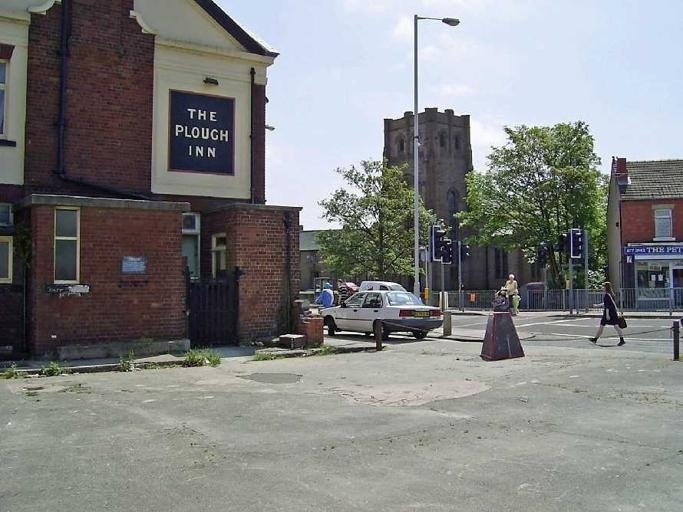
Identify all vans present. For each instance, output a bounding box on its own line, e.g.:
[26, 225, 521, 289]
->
[359, 281, 413, 306]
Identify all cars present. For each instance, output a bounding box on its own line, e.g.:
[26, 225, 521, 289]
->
[318, 290, 444, 341]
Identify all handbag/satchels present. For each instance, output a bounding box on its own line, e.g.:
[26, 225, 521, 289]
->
[617, 315, 627, 329]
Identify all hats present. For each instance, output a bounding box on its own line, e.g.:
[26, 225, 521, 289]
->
[325, 283, 333, 289]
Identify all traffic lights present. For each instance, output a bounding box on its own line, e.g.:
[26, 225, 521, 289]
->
[557, 228, 582, 259]
[431, 225, 452, 266]
[461, 242, 471, 261]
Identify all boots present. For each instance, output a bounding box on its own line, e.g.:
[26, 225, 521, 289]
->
[588, 336, 599, 344]
[617, 337, 625, 346]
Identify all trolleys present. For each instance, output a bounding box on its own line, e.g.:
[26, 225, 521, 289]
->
[493, 288, 513, 318]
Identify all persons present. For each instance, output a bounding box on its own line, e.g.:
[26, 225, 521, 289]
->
[511, 289, 521, 315]
[335, 279, 349, 305]
[587, 282, 625, 346]
[314, 283, 334, 308]
[504, 274, 518, 315]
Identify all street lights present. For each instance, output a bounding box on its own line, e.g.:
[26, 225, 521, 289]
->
[412, 13, 460, 301]
